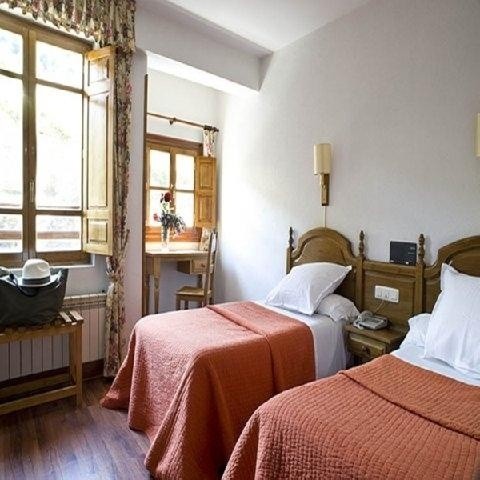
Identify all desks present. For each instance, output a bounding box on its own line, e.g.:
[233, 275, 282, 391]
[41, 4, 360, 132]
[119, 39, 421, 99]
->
[145, 248, 215, 314]
[1, 309, 85, 416]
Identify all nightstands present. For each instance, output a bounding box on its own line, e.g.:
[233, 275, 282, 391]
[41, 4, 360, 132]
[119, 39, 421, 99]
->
[344, 323, 407, 368]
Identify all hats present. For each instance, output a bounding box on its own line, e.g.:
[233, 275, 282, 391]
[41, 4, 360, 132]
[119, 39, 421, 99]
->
[14, 257, 63, 289]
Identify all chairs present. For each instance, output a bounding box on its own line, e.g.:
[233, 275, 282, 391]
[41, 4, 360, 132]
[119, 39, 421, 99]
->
[174, 232, 218, 310]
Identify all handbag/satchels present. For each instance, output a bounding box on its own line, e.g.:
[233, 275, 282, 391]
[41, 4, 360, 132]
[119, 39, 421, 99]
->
[0, 263, 69, 328]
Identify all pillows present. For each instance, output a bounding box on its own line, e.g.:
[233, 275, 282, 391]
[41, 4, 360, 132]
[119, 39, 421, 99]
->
[399, 311, 431, 349]
[264, 260, 354, 316]
[317, 296, 360, 323]
[422, 261, 480, 378]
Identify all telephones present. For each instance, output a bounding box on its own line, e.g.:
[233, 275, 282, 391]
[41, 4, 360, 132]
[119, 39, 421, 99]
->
[354, 310, 388, 330]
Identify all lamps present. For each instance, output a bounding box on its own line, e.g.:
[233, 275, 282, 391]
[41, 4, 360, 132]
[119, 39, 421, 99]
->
[313, 142, 333, 207]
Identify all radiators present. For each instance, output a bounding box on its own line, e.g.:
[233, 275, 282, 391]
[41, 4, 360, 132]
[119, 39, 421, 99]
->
[0, 292, 111, 382]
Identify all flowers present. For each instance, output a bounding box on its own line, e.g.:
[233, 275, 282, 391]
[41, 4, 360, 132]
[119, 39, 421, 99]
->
[153, 183, 187, 236]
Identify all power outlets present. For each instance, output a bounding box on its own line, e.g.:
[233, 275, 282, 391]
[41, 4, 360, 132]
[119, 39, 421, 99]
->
[374, 284, 399, 304]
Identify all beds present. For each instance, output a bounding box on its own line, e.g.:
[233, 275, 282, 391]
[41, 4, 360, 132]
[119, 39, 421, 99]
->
[219, 232, 480, 480]
[94, 226, 366, 480]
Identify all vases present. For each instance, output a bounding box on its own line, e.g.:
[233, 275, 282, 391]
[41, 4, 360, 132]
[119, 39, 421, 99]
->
[160, 225, 171, 250]
